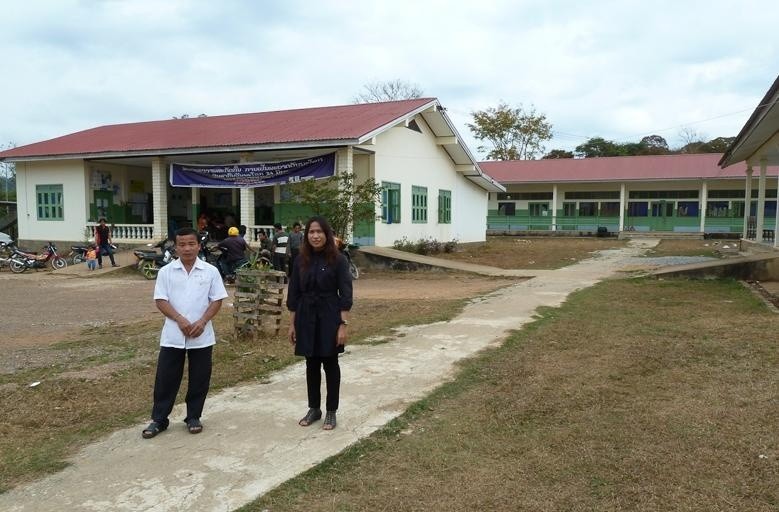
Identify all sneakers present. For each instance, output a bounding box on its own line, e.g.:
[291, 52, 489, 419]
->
[112, 264, 120, 267]
[98, 264, 103, 269]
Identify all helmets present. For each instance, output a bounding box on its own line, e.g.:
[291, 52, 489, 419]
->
[228, 227, 239, 236]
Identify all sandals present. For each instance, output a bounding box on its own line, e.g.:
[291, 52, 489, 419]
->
[185, 416, 202, 434]
[299, 408, 322, 425]
[142, 421, 167, 438]
[324, 411, 336, 430]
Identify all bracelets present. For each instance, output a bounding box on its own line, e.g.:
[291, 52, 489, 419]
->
[174, 313, 181, 322]
[340, 319, 348, 325]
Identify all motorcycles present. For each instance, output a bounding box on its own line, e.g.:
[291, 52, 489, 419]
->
[71, 243, 117, 265]
[7, 241, 67, 274]
[338, 244, 359, 279]
[133, 230, 211, 280]
[212, 246, 251, 283]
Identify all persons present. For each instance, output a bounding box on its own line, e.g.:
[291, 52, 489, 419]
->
[199, 210, 236, 241]
[95, 218, 120, 269]
[285, 216, 354, 430]
[258, 231, 274, 262]
[239, 225, 257, 267]
[216, 226, 246, 283]
[85, 244, 99, 271]
[142, 227, 228, 439]
[286, 222, 305, 278]
[271, 222, 290, 284]
